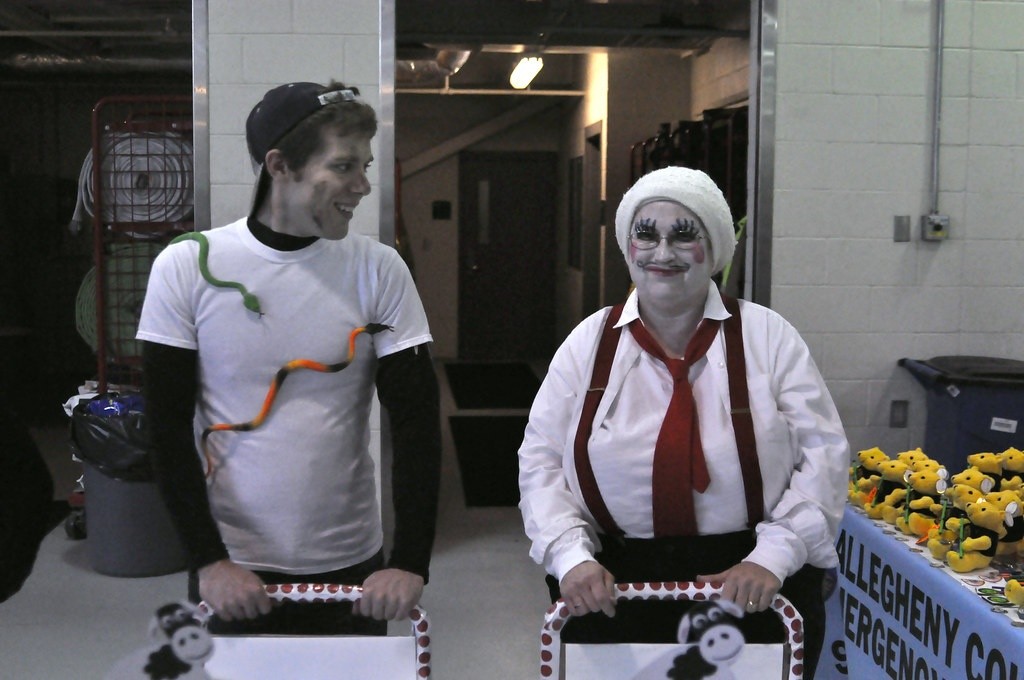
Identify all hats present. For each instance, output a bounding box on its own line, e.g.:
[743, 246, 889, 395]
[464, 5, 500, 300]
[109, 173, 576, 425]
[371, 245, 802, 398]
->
[246, 82, 355, 165]
[615, 165, 735, 276]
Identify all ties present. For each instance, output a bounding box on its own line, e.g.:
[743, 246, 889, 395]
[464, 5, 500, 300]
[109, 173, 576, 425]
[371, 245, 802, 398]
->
[626, 319, 722, 539]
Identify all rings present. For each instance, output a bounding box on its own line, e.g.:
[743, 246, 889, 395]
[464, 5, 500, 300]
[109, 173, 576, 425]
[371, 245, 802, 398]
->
[748, 601, 753, 606]
[574, 603, 581, 608]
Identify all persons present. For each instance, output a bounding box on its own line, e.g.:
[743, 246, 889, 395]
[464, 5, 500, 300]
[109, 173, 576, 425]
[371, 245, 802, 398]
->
[133, 80, 443, 636]
[516, 166, 850, 680]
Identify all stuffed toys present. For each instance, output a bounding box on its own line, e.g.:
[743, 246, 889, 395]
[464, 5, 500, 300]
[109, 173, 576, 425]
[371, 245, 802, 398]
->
[927, 501, 1014, 573]
[881, 469, 947, 534]
[845, 436, 1024, 616]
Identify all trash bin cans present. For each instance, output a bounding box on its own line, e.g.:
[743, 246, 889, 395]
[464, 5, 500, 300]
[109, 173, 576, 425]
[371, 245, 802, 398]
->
[69, 391, 188, 579]
[897, 355, 1024, 471]
